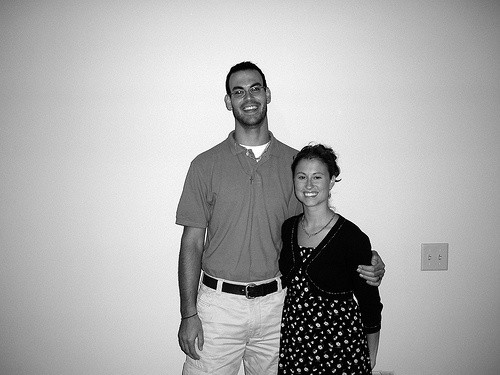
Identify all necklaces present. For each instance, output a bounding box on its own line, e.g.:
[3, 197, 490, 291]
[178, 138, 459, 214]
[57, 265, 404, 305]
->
[302, 214, 335, 239]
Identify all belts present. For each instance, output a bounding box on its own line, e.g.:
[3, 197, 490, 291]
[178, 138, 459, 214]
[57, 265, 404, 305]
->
[202, 273, 287, 299]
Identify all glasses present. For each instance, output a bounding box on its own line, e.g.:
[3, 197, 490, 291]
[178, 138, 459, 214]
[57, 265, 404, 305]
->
[230, 87, 265, 98]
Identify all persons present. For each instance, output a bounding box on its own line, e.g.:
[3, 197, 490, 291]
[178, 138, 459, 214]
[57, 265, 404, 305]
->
[278, 144, 383, 375]
[176, 61, 385, 375]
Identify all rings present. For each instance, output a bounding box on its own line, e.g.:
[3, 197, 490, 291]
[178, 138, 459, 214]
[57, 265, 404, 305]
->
[379, 275, 382, 281]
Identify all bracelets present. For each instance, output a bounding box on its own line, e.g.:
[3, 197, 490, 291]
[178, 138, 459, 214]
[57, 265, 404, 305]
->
[181, 313, 197, 320]
